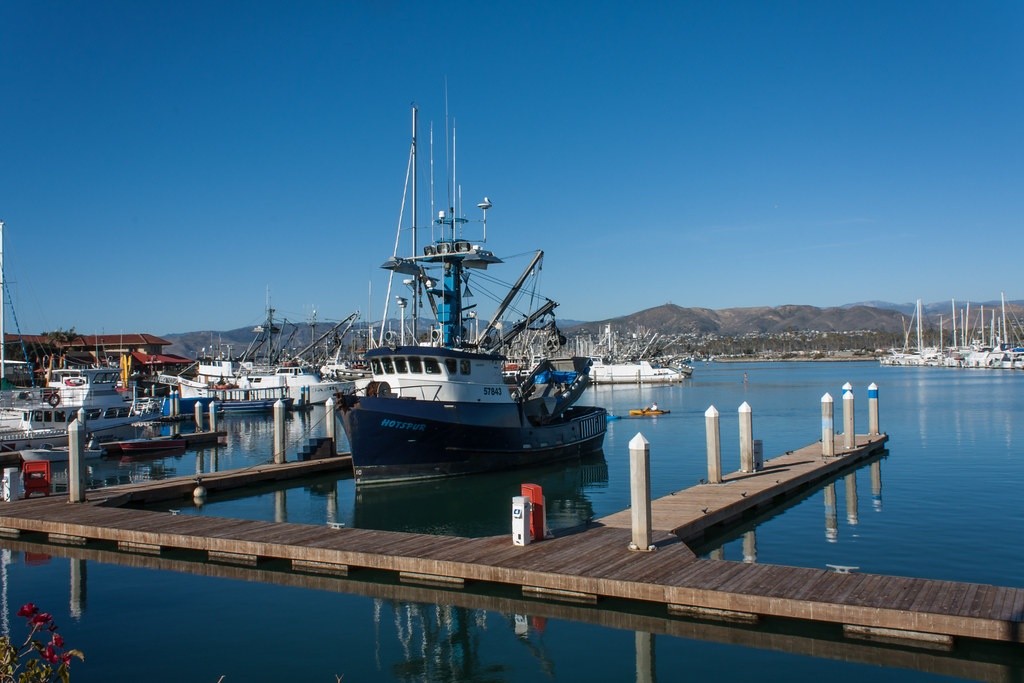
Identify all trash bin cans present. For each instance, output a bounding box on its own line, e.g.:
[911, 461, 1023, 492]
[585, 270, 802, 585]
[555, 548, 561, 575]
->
[512, 496, 530, 546]
[2, 468, 18, 502]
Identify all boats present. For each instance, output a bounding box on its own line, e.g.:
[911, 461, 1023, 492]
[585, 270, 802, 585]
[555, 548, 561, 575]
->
[1, 255, 701, 468]
[325, 76, 611, 489]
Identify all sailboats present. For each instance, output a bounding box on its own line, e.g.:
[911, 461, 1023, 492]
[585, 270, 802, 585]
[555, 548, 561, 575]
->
[878, 291, 1024, 371]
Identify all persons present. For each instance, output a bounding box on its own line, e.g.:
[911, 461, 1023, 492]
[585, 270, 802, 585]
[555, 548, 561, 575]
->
[643, 402, 658, 411]
